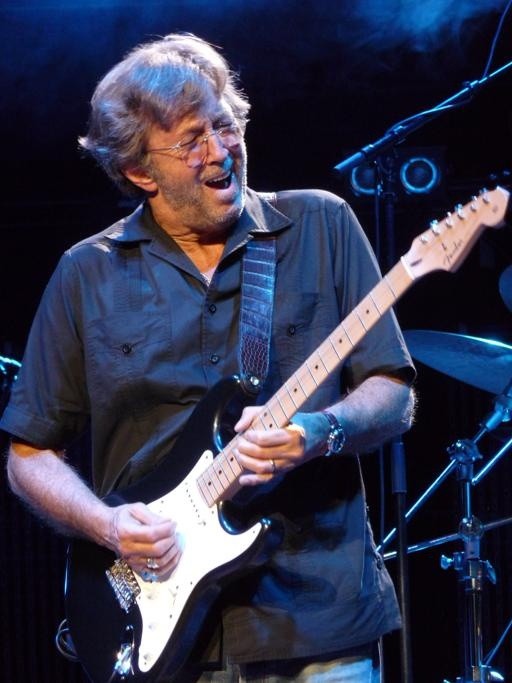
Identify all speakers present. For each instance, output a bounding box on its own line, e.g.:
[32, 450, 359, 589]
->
[342, 144, 448, 204]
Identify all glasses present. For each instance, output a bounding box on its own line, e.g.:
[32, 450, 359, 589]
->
[143, 125, 243, 169]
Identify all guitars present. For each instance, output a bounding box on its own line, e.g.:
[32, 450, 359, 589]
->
[64, 186, 508, 682]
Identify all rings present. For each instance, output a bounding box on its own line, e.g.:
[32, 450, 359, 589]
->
[141, 569, 158, 583]
[268, 458, 276, 473]
[146, 556, 159, 569]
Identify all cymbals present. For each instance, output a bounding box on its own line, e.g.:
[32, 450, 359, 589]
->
[403, 330, 511, 394]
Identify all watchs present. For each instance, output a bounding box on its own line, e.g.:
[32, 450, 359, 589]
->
[320, 409, 346, 458]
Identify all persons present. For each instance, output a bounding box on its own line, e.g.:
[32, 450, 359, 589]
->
[2, 31, 419, 683]
